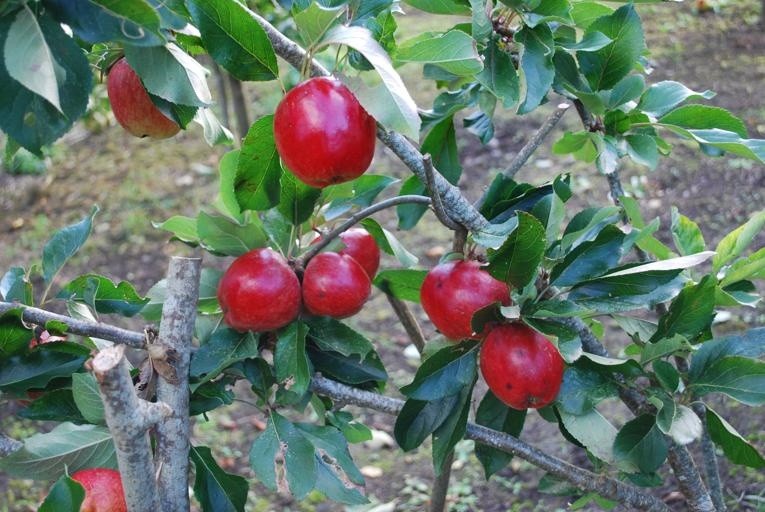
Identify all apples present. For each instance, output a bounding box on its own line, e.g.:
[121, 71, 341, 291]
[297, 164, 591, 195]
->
[39, 469, 128, 511]
[420, 259, 512, 342]
[104, 55, 180, 140]
[272, 75, 376, 189]
[301, 252, 373, 318]
[480, 319, 563, 411]
[14, 328, 103, 405]
[217, 248, 301, 334]
[309, 227, 380, 280]
